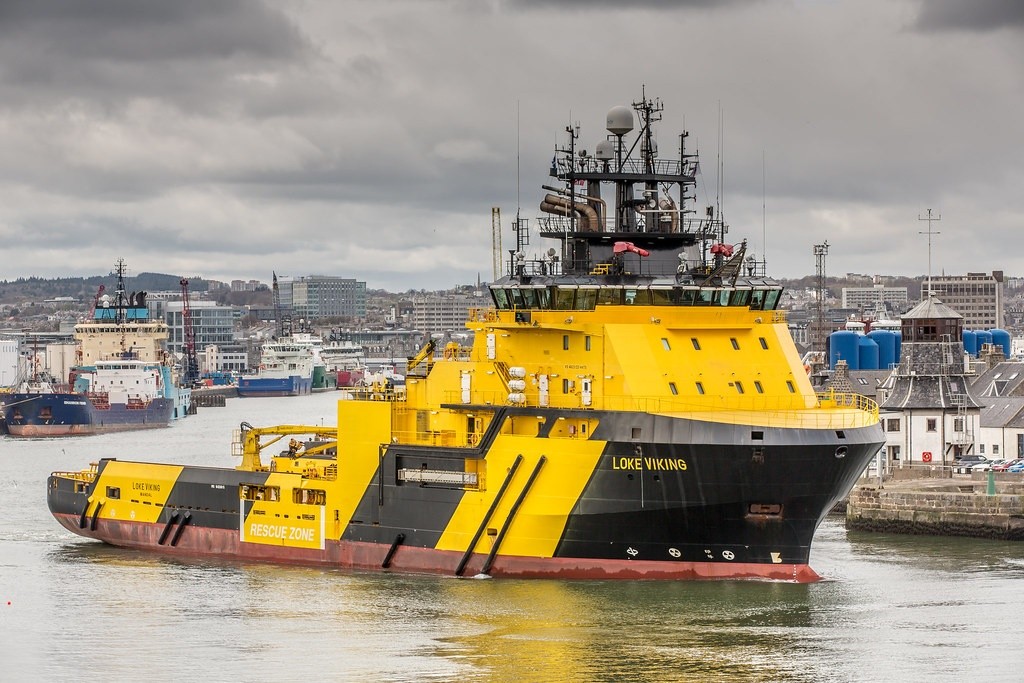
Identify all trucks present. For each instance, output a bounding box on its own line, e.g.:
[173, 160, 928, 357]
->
[204, 372, 235, 385]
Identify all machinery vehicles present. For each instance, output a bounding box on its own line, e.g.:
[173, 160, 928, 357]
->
[269, 269, 287, 338]
[490, 207, 504, 280]
[177, 277, 205, 388]
[230, 419, 337, 482]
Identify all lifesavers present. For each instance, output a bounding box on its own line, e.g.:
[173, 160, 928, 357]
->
[923, 453, 931, 461]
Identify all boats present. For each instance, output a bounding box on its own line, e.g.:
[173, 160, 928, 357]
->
[293, 333, 338, 393]
[69, 281, 192, 419]
[374, 365, 405, 385]
[45, 84, 886, 587]
[318, 341, 366, 390]
[237, 335, 316, 395]
[0, 348, 176, 439]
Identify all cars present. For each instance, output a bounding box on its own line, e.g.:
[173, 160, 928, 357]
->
[971, 459, 1003, 472]
[993, 459, 1022, 472]
[1008, 460, 1024, 472]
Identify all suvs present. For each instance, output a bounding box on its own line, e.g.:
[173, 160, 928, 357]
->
[951, 455, 987, 474]
